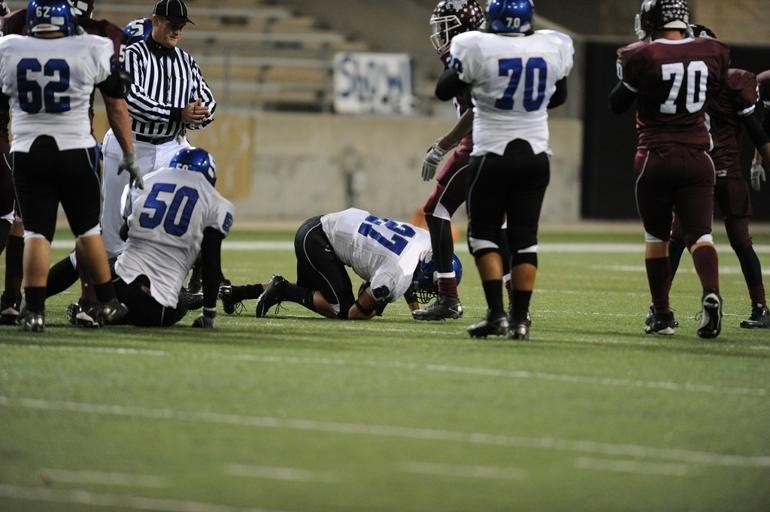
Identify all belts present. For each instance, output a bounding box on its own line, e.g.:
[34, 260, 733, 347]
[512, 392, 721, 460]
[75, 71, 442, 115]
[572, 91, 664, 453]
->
[136, 135, 174, 145]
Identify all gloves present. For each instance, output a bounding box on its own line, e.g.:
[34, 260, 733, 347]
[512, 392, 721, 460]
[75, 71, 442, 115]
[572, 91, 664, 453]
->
[191, 314, 214, 328]
[116, 157, 144, 190]
[750, 159, 766, 193]
[421, 144, 445, 181]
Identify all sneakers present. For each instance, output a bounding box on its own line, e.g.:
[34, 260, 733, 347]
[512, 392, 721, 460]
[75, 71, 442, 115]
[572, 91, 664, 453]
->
[645, 303, 679, 327]
[644, 312, 675, 335]
[411, 296, 463, 321]
[466, 308, 510, 338]
[1, 278, 248, 332]
[508, 309, 531, 341]
[256, 273, 288, 318]
[739, 306, 770, 328]
[695, 288, 723, 339]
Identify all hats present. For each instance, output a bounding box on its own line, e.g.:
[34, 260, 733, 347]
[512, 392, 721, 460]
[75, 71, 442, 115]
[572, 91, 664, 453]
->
[153, 1, 196, 25]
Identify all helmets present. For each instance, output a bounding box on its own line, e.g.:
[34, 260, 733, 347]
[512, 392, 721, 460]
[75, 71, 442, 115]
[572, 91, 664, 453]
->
[70, 0, 94, 20]
[483, 1, 533, 32]
[169, 147, 217, 186]
[689, 24, 718, 40]
[429, 0, 486, 59]
[410, 249, 463, 305]
[634, 0, 689, 41]
[26, 0, 77, 37]
[123, 17, 152, 39]
[0, 1, 10, 30]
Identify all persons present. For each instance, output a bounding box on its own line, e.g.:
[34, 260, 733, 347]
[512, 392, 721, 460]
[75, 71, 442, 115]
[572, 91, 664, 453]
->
[749, 68, 770, 191]
[0, 0, 233, 333]
[411, 0, 493, 319]
[432, 0, 578, 339]
[218, 206, 462, 322]
[613, 0, 734, 341]
[643, 20, 770, 333]
